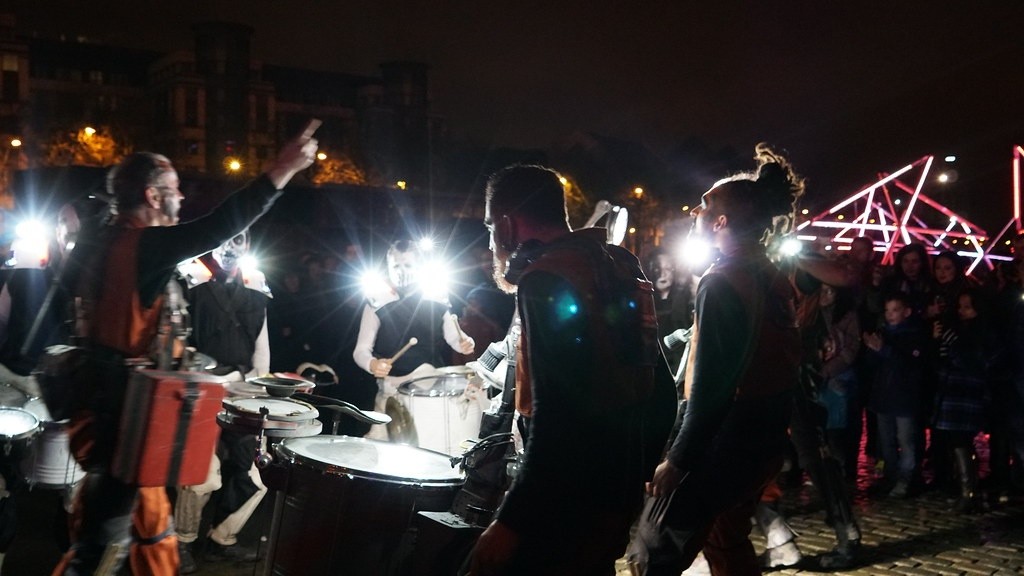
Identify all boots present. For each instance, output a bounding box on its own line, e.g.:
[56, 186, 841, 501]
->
[981, 434, 1013, 494]
[947, 446, 988, 516]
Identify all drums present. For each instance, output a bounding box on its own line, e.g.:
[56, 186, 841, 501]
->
[0, 405, 40, 464]
[397, 370, 488, 459]
[263, 434, 470, 576]
[20, 395, 88, 488]
[213, 393, 324, 438]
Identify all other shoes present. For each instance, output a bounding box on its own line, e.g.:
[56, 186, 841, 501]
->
[877, 475, 896, 490]
[758, 540, 802, 569]
[890, 478, 918, 498]
[178, 541, 196, 573]
[817, 540, 865, 568]
[203, 538, 260, 563]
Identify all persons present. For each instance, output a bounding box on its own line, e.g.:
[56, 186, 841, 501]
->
[1, 118, 1024, 575]
[625, 143, 802, 575]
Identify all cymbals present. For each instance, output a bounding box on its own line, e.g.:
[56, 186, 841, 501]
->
[292, 391, 393, 426]
[250, 376, 317, 397]
